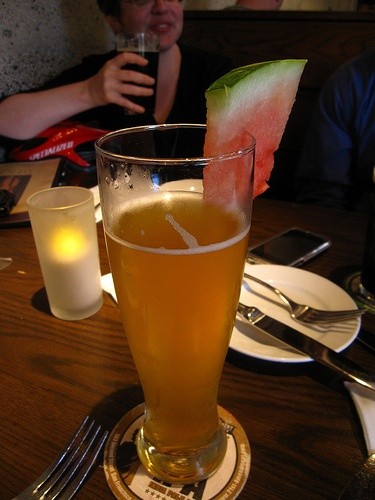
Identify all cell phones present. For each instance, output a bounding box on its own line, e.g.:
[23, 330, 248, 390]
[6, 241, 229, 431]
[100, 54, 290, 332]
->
[251, 227, 333, 266]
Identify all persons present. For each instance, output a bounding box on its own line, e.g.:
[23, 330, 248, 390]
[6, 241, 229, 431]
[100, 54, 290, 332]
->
[0, 0, 274, 210]
[311, 36, 375, 196]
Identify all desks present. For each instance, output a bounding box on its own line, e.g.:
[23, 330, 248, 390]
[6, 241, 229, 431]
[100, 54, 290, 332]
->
[0, 197, 375, 500]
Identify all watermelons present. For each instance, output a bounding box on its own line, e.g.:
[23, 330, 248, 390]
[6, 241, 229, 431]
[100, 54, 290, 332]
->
[202, 58, 308, 206]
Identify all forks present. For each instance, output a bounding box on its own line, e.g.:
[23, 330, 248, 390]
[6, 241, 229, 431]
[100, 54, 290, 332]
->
[8, 415, 109, 500]
[244, 272, 367, 324]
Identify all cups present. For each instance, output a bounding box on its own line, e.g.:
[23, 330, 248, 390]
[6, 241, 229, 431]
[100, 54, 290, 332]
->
[96, 122, 255, 483]
[27, 186, 104, 321]
[115, 31, 159, 116]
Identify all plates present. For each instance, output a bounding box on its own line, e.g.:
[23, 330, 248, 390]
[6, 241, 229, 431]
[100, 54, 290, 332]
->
[228, 264, 360, 363]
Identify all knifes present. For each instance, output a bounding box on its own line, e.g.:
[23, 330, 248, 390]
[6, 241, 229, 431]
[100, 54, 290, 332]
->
[236, 302, 375, 392]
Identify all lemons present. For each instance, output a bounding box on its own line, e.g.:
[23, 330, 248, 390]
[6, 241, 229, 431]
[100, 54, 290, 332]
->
[46, 222, 87, 261]
